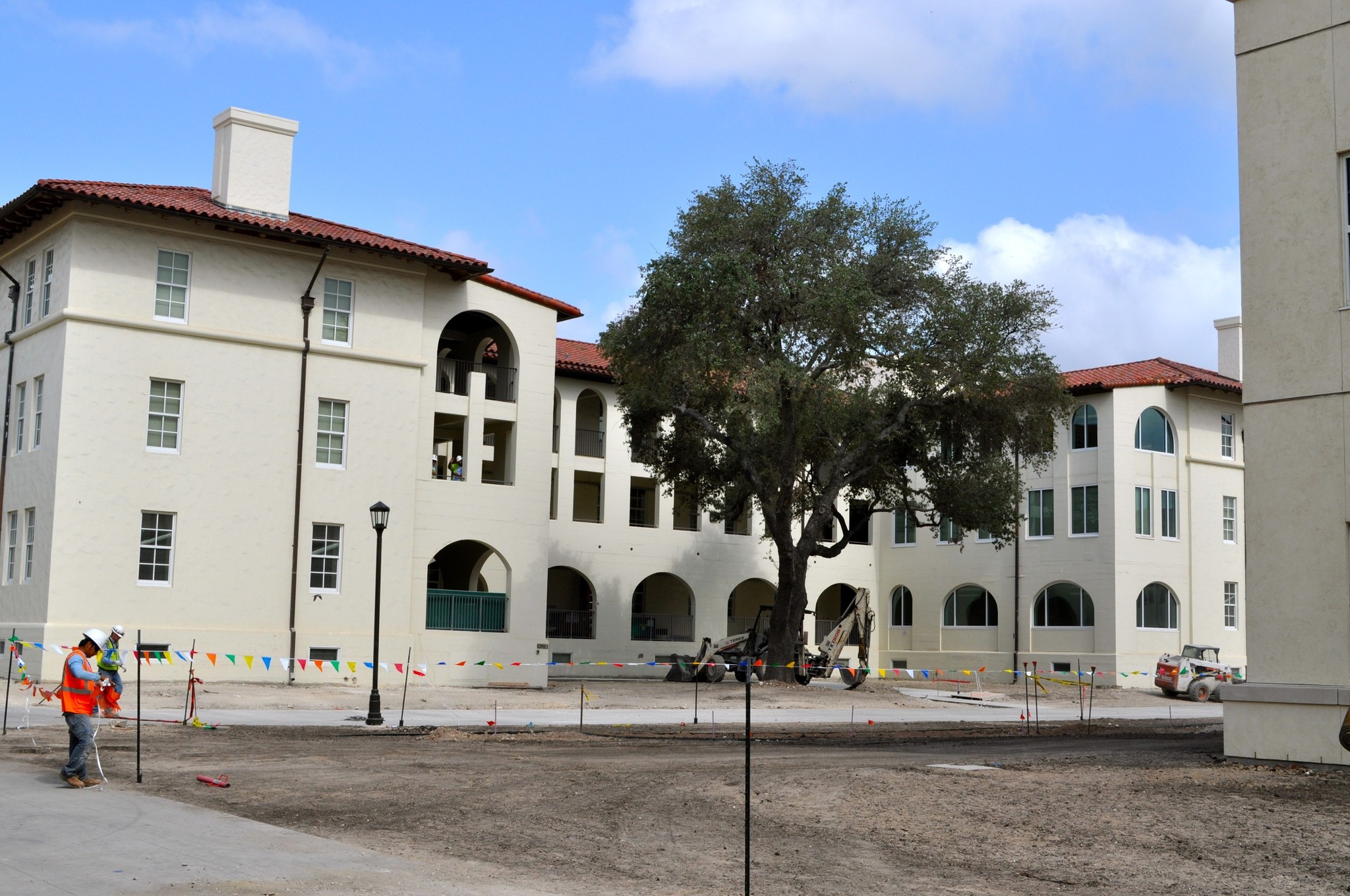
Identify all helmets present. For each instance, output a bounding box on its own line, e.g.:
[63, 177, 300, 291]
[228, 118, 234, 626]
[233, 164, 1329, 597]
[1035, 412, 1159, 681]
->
[83, 629, 107, 653]
[433, 454, 438, 461]
[112, 625, 124, 638]
[456, 455, 462, 462]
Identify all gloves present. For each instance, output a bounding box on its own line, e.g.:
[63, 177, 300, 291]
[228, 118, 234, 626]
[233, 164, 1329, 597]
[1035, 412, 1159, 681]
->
[113, 660, 123, 666]
[121, 665, 126, 673]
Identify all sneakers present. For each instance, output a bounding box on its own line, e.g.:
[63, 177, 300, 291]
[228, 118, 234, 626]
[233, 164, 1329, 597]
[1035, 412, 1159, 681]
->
[82, 778, 101, 786]
[59, 772, 85, 788]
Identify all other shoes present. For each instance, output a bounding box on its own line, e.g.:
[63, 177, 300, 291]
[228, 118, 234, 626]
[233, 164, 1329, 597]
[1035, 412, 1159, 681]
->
[104, 706, 119, 717]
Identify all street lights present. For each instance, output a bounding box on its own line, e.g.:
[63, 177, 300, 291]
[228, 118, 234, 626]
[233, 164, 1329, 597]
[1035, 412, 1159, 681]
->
[365, 501, 394, 725]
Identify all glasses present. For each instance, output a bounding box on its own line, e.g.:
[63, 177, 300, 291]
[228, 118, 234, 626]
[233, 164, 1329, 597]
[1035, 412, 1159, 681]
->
[433, 460, 436, 462]
[94, 647, 100, 652]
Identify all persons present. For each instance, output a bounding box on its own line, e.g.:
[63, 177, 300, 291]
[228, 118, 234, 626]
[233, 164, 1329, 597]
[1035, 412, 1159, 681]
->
[432, 454, 438, 479]
[90, 625, 126, 718]
[59, 629, 118, 789]
[448, 455, 463, 481]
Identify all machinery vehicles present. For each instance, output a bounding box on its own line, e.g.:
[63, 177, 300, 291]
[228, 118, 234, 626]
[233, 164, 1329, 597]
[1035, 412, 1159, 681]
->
[663, 585, 878, 691]
[1153, 644, 1246, 702]
